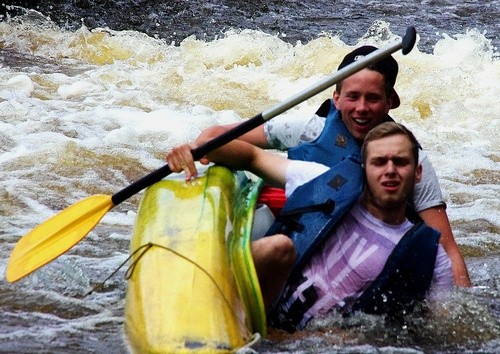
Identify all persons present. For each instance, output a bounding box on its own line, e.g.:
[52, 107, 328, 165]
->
[192, 45, 474, 292]
[165, 122, 456, 346]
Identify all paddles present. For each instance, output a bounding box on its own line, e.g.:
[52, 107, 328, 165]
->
[6, 25, 416, 283]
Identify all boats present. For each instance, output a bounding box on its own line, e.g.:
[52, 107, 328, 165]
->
[123, 162, 287, 354]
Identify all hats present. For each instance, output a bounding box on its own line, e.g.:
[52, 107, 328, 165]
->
[338, 46, 399, 110]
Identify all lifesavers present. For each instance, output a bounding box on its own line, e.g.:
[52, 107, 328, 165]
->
[264, 158, 441, 331]
[287, 99, 422, 168]
